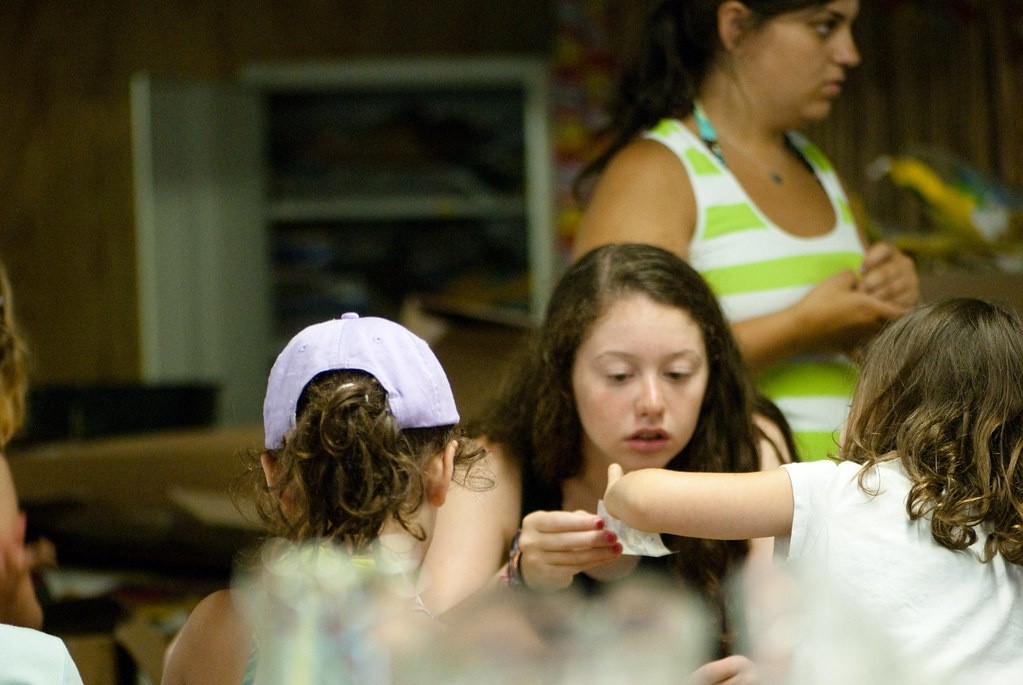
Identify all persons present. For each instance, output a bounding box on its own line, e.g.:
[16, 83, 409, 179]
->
[567, 0, 923, 570]
[603, 297, 1023, 684]
[381, 242, 786, 684]
[0, 456, 87, 685]
[1, 262, 45, 628]
[160, 310, 501, 684]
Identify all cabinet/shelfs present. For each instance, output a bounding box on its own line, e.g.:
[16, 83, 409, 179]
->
[133, 56, 554, 417]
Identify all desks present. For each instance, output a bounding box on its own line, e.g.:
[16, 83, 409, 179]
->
[3, 427, 265, 572]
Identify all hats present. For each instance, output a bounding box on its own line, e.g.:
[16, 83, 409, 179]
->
[266, 313, 462, 447]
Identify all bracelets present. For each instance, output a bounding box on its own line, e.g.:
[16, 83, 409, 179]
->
[507, 550, 526, 593]
